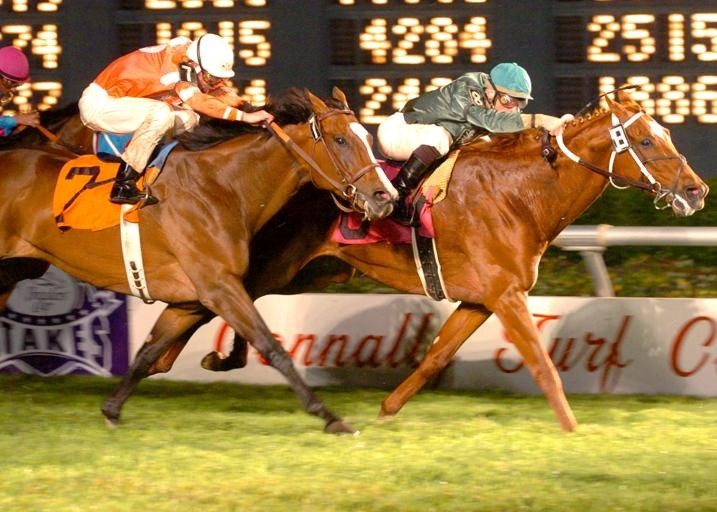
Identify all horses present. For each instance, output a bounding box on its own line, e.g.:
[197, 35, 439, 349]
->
[143, 89, 710, 433]
[0, 101, 98, 312]
[0, 86, 399, 434]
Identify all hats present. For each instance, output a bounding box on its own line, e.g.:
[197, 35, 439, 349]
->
[487, 61, 534, 100]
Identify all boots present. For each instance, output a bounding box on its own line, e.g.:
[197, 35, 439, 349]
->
[109, 158, 159, 207]
[381, 152, 428, 226]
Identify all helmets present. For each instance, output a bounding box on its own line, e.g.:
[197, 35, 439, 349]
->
[0, 45, 32, 84]
[186, 33, 236, 77]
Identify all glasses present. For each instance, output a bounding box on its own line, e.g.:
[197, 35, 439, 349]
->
[497, 94, 529, 109]
[202, 71, 222, 84]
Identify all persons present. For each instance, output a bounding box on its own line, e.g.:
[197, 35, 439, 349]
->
[374, 61, 575, 230]
[0, 44, 43, 138]
[76, 33, 274, 205]
